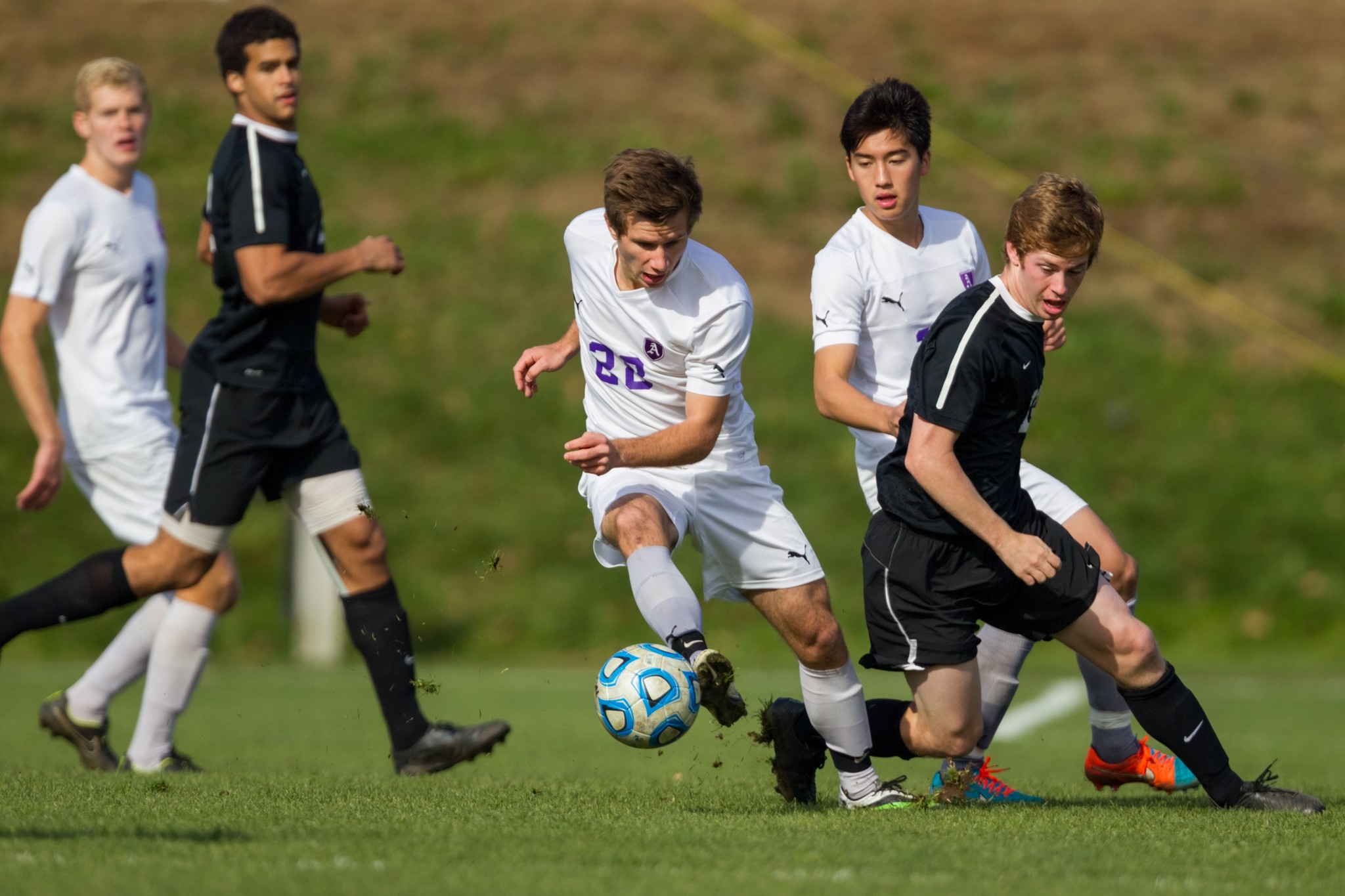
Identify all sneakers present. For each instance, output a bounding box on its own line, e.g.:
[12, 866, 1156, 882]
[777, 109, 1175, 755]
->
[693, 648, 746, 726]
[931, 757, 1043, 805]
[1084, 739, 1201, 795]
[1209, 780, 1326, 813]
[39, 689, 119, 771]
[391, 719, 511, 775]
[839, 783, 939, 809]
[118, 751, 211, 774]
[767, 697, 827, 805]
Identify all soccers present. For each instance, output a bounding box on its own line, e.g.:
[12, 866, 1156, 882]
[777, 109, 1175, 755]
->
[594, 643, 701, 748]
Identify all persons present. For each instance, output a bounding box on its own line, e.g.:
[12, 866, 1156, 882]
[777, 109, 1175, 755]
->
[1, 55, 248, 777]
[511, 146, 948, 810]
[1, 5, 511, 781]
[809, 76, 1204, 820]
[759, 172, 1330, 822]
